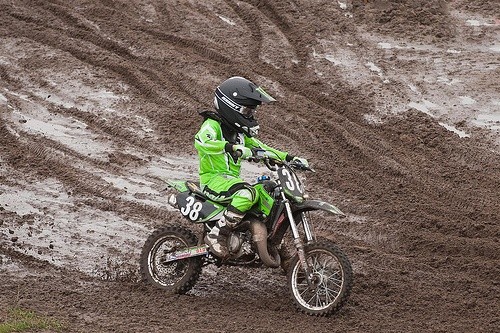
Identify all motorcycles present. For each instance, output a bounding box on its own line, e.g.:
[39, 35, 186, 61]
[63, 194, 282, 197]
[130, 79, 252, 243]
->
[139, 146, 354, 318]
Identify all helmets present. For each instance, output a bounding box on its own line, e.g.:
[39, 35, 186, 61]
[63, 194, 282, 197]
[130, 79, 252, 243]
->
[214, 77, 276, 137]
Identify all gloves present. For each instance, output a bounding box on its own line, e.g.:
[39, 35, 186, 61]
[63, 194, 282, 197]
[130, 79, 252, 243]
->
[293, 156, 309, 168]
[234, 145, 253, 160]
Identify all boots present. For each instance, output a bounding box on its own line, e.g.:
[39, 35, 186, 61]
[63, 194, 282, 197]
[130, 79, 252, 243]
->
[204, 206, 245, 260]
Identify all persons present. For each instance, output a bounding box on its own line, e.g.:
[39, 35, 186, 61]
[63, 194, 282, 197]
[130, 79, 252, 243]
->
[194, 77, 309, 257]
